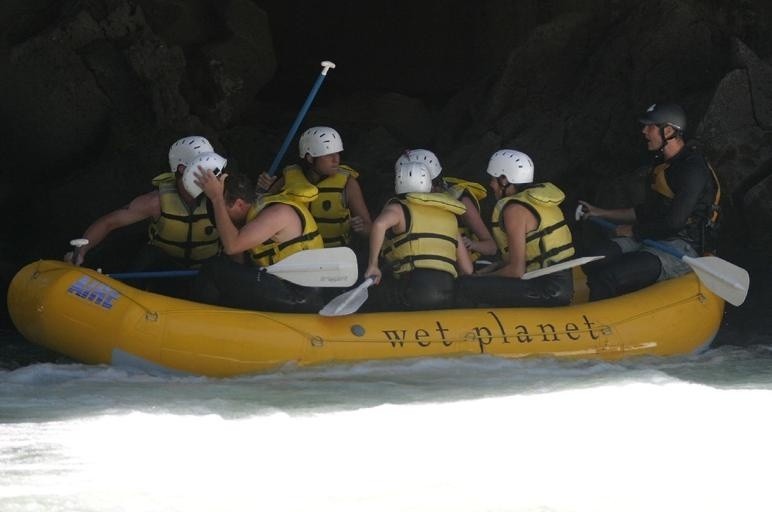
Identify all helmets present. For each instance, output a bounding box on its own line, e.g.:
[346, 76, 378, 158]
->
[395, 149, 442, 195]
[487, 150, 534, 183]
[168, 136, 228, 199]
[298, 126, 344, 159]
[639, 102, 689, 133]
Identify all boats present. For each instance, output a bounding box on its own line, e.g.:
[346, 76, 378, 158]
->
[7, 231, 721, 380]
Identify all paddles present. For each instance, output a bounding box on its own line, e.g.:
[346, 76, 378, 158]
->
[319, 275, 377, 316]
[104, 246, 358, 288]
[575, 201, 749, 307]
[521, 255, 605, 281]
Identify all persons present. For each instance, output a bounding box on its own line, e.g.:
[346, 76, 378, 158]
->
[64, 134, 244, 271]
[253, 125, 372, 262]
[575, 99, 724, 304]
[395, 149, 497, 269]
[191, 160, 331, 314]
[452, 148, 576, 309]
[350, 160, 475, 314]
